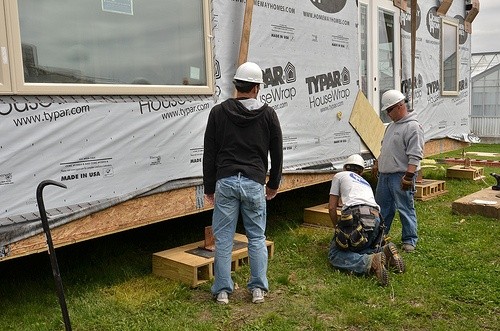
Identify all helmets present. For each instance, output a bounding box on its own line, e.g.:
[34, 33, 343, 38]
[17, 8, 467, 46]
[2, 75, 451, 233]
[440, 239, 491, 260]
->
[343, 154, 365, 170]
[234, 62, 264, 84]
[380, 89, 406, 111]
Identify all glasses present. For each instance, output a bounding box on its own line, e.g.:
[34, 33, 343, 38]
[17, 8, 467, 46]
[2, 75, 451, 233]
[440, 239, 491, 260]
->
[386, 104, 402, 113]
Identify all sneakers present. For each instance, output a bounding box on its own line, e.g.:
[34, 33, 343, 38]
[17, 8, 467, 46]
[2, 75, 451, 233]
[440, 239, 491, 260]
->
[216, 291, 229, 306]
[251, 288, 267, 304]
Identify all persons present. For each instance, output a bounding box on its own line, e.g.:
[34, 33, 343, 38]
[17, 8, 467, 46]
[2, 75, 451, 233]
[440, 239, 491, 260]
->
[202, 62, 283, 305]
[374, 90, 425, 252]
[328, 154, 405, 287]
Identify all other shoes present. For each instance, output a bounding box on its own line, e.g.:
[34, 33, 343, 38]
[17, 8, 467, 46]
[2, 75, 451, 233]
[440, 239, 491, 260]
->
[371, 252, 389, 288]
[403, 243, 414, 253]
[382, 242, 405, 275]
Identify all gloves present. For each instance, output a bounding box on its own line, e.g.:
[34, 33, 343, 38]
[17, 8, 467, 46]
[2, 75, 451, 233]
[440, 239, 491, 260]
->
[373, 159, 379, 179]
[400, 170, 416, 192]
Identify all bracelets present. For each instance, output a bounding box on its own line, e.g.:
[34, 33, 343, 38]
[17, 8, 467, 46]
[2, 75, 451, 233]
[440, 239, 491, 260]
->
[334, 223, 339, 229]
